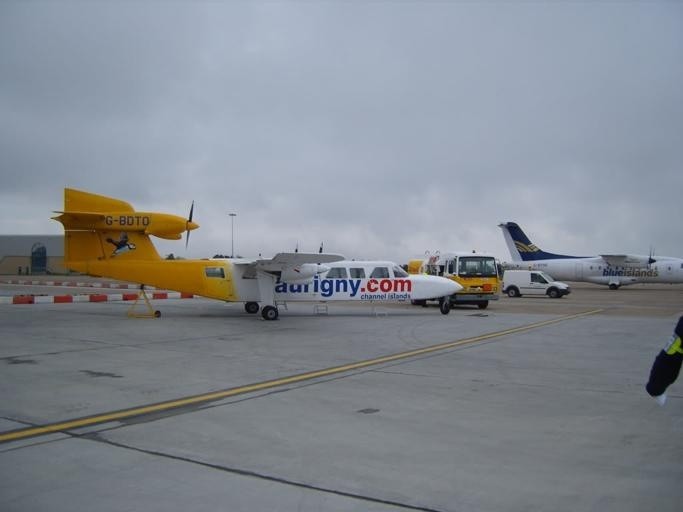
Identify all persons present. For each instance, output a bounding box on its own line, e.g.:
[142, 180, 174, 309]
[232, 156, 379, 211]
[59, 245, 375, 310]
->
[643, 313, 683, 405]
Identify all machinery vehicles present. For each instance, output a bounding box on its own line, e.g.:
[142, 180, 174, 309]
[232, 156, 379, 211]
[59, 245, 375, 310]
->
[405, 249, 504, 310]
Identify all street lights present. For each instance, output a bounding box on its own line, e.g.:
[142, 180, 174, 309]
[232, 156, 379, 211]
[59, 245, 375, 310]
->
[228, 211, 237, 256]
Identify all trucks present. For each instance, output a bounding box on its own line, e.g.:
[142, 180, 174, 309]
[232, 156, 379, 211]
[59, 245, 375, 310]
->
[501, 269, 572, 300]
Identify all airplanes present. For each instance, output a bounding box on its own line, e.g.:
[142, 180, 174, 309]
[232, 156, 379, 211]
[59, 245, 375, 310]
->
[49, 185, 467, 320]
[494, 219, 683, 293]
